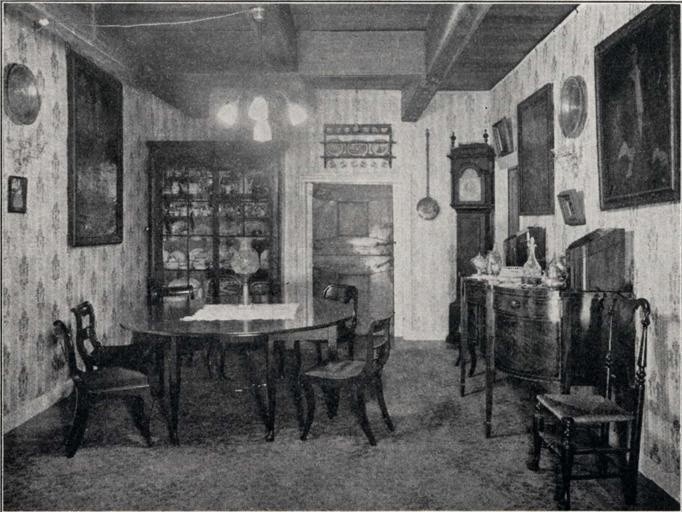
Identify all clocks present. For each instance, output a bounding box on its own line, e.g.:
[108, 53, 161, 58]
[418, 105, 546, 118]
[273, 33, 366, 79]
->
[446, 129, 495, 353]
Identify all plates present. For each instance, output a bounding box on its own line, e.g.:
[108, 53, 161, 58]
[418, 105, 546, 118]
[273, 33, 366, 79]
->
[167, 169, 272, 298]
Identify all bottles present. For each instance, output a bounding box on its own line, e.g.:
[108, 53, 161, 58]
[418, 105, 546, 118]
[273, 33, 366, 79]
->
[468, 232, 566, 285]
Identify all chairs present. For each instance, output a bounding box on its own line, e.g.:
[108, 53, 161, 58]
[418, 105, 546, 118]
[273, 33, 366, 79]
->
[70, 301, 158, 404]
[294, 283, 358, 377]
[147, 274, 284, 381]
[54, 320, 150, 458]
[524, 287, 655, 510]
[300, 311, 394, 446]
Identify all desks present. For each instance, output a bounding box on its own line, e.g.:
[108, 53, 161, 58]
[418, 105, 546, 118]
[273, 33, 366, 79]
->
[120, 296, 355, 441]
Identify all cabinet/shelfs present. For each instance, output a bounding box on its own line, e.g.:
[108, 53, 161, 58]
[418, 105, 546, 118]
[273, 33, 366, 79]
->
[144, 133, 288, 304]
[459, 278, 563, 441]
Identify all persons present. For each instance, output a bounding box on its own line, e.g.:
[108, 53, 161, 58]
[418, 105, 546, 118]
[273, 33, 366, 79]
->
[605, 35, 671, 196]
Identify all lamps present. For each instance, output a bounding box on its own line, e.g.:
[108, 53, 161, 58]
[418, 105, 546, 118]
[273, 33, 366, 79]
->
[211, 7, 309, 143]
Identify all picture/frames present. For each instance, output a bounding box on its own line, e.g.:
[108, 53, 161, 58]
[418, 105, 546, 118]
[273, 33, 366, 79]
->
[66, 45, 124, 248]
[592, 4, 680, 212]
[558, 189, 585, 226]
[493, 117, 514, 158]
[8, 174, 28, 214]
[516, 79, 556, 218]
